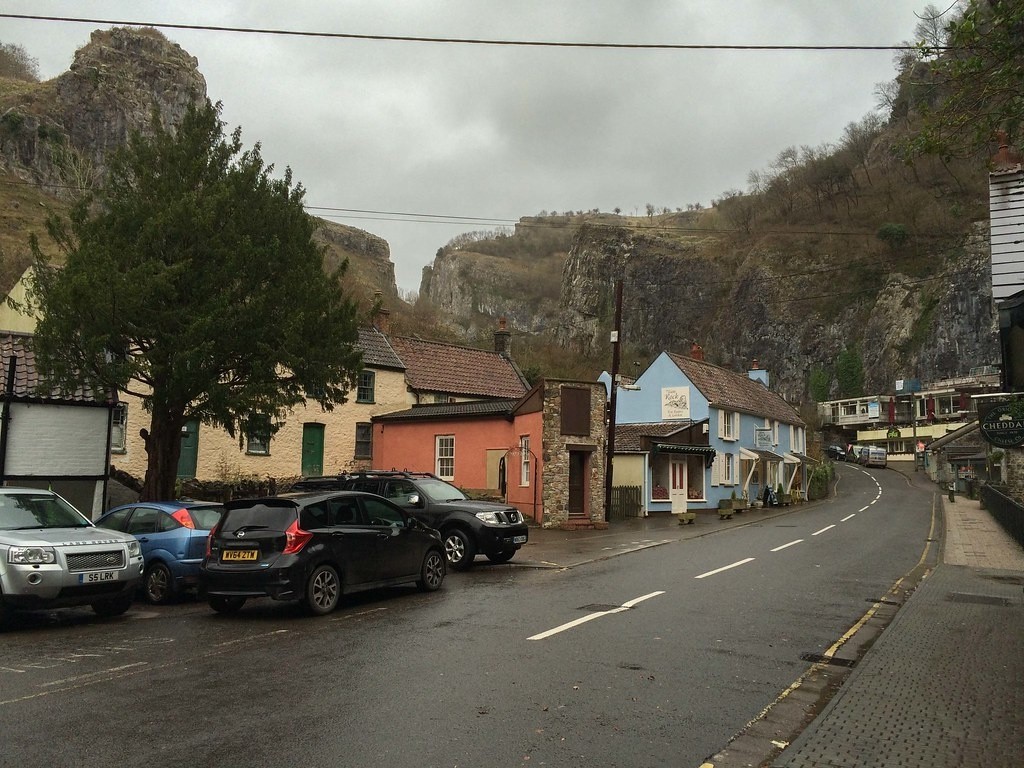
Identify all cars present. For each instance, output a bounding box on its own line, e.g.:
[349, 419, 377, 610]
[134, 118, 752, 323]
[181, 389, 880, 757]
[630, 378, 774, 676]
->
[200, 490, 448, 617]
[94, 499, 224, 605]
[845, 445, 864, 462]
[828, 444, 845, 460]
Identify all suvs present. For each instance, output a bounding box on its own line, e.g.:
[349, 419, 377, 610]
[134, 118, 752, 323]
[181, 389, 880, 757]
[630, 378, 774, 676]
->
[289, 467, 529, 570]
[0, 485, 144, 617]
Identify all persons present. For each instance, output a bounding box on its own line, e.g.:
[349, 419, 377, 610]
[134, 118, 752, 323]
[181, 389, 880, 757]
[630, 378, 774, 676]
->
[948, 478, 956, 502]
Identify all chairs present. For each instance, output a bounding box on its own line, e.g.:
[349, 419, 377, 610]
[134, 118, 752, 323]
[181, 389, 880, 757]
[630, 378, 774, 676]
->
[336, 506, 357, 523]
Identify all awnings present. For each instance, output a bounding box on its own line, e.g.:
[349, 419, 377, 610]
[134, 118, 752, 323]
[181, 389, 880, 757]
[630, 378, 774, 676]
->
[740, 446, 785, 493]
[783, 453, 819, 500]
[650, 442, 717, 467]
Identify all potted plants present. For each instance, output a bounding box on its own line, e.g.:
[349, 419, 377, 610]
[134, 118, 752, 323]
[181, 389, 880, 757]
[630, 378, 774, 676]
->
[720, 490, 747, 510]
[753, 495, 763, 506]
[777, 483, 785, 507]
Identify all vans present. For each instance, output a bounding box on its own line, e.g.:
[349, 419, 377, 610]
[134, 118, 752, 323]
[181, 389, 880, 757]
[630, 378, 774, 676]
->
[858, 447, 887, 468]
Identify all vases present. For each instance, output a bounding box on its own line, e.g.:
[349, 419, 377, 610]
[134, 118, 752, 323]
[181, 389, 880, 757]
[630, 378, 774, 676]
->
[718, 509, 733, 515]
[678, 513, 696, 520]
[776, 494, 792, 503]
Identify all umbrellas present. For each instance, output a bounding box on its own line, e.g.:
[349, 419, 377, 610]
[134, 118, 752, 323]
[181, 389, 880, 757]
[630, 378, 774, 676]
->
[960, 391, 966, 423]
[927, 393, 934, 424]
[888, 397, 895, 425]
